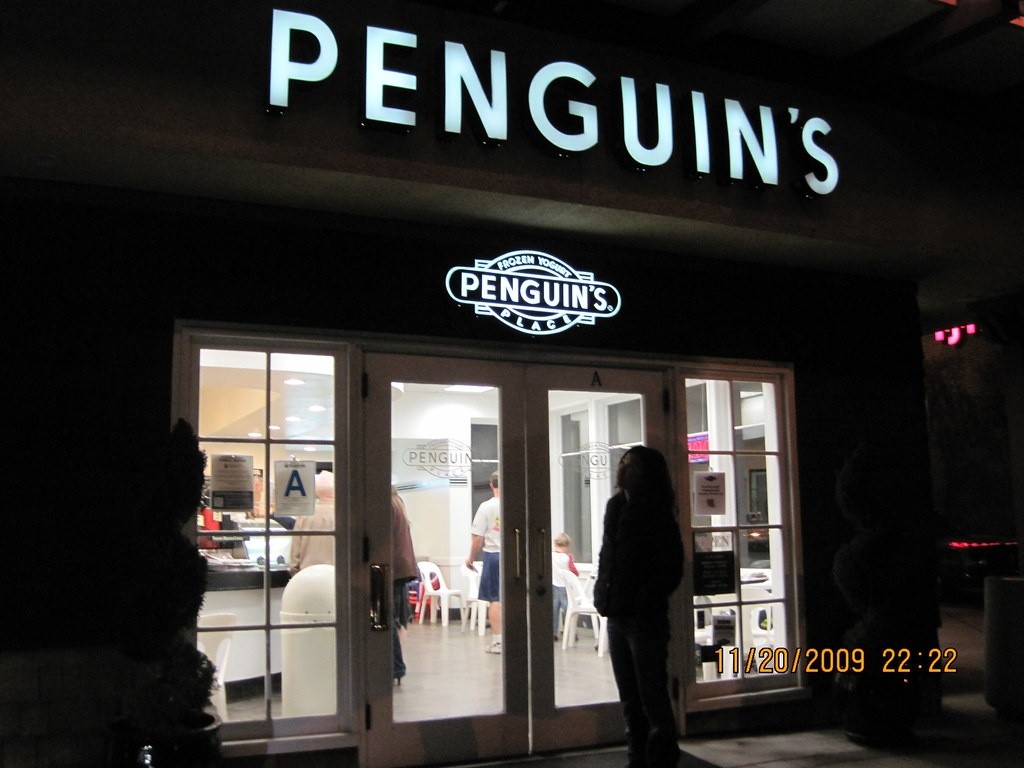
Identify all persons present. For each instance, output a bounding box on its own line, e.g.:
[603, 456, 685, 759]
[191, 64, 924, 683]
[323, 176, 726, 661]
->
[392, 486, 418, 684]
[552, 533, 578, 641]
[289, 470, 335, 577]
[593, 446, 685, 768]
[467, 471, 502, 653]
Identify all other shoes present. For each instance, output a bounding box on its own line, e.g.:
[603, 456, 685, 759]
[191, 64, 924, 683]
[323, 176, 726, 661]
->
[486, 640, 502, 654]
[575, 633, 579, 642]
[554, 635, 560, 642]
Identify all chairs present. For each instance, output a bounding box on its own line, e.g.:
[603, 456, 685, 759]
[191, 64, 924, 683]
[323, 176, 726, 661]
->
[406, 560, 491, 636]
[734, 587, 778, 676]
[559, 565, 608, 659]
[196, 614, 240, 723]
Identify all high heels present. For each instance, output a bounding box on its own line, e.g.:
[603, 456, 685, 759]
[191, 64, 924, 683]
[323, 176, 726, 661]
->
[394, 666, 406, 685]
